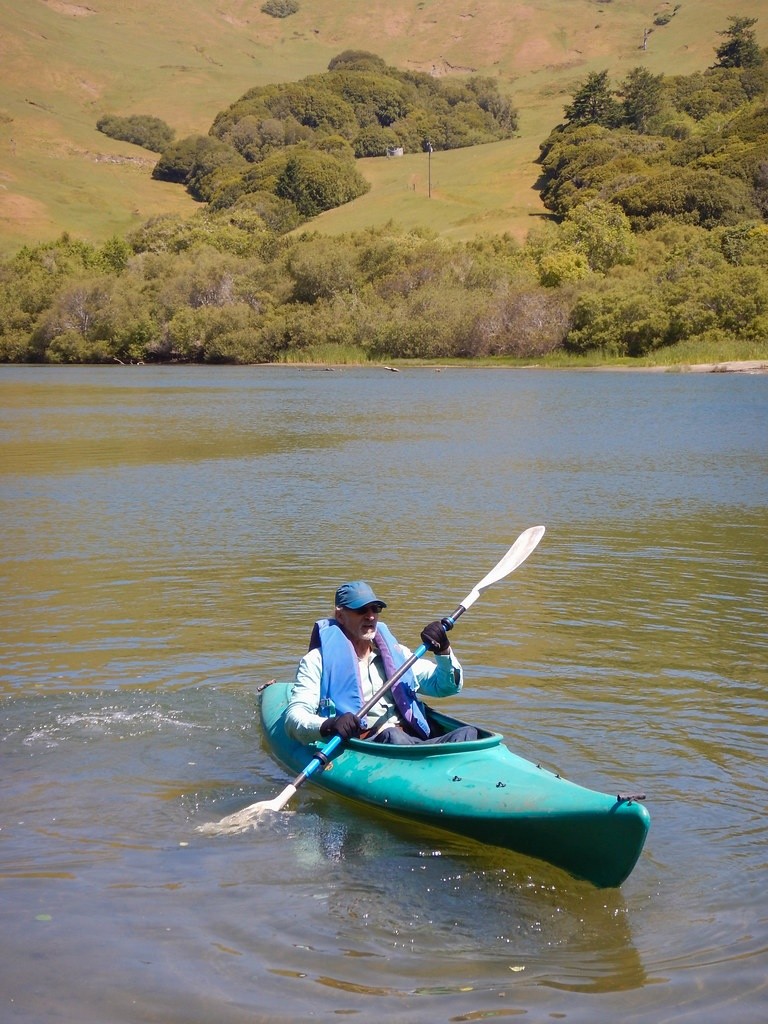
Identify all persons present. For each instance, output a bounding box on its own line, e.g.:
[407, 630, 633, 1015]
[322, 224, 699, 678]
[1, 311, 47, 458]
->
[284, 581, 479, 746]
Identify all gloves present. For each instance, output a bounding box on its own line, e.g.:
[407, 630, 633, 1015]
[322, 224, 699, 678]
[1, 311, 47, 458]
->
[320, 711, 363, 743]
[420, 621, 450, 654]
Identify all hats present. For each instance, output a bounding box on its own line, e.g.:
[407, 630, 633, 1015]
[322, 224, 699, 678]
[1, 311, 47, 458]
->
[334, 580, 387, 609]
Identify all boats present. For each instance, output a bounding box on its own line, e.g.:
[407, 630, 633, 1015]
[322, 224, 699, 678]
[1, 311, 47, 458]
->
[259, 680, 652, 890]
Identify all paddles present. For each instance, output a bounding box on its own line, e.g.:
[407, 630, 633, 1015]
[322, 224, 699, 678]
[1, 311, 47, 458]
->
[219, 525, 546, 823]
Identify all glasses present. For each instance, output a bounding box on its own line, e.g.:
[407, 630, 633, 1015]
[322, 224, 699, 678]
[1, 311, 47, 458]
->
[351, 604, 384, 613]
